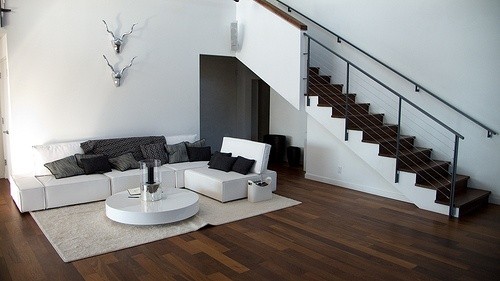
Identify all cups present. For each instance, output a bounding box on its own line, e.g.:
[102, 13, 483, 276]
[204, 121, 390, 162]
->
[138, 158, 162, 203]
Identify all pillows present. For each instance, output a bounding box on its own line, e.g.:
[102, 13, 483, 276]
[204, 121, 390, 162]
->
[44, 139, 256, 179]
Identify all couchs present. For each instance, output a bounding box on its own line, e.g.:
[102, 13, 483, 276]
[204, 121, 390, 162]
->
[9, 135, 210, 213]
[183, 136, 278, 203]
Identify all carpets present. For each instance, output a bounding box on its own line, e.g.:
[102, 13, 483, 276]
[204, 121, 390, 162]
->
[29, 185, 303, 262]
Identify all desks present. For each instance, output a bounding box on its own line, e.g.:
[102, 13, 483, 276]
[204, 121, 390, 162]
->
[105, 187, 200, 226]
